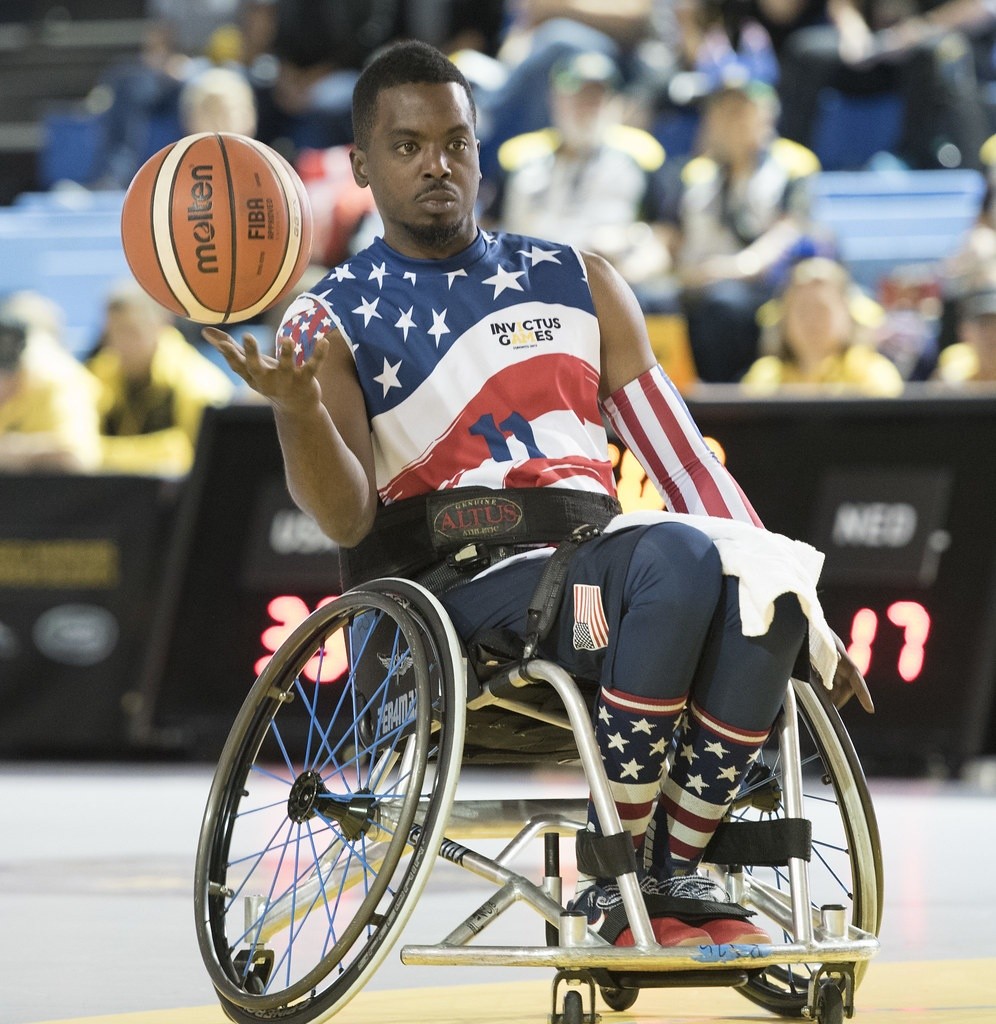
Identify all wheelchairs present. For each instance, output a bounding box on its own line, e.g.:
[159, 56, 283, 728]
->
[190, 530, 888, 1024]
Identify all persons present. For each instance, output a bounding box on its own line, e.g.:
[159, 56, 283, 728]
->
[200, 38, 811, 962]
[1, 0, 993, 458]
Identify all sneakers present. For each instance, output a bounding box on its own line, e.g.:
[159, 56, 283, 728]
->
[641, 876, 772, 980]
[566, 884, 717, 976]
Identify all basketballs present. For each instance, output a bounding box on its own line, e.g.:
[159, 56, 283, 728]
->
[115, 129, 316, 332]
